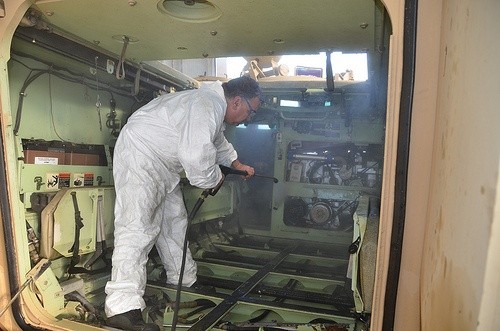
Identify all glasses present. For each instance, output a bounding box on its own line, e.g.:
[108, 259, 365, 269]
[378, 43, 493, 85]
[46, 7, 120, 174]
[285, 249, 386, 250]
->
[243, 96, 257, 121]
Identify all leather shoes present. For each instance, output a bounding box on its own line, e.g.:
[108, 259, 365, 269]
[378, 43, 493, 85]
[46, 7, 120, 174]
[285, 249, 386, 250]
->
[105, 309, 161, 331]
[183, 281, 216, 295]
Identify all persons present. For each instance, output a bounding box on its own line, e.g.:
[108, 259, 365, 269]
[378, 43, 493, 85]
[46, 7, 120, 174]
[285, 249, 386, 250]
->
[102, 76, 263, 331]
